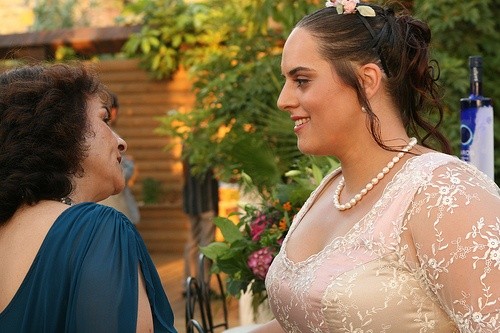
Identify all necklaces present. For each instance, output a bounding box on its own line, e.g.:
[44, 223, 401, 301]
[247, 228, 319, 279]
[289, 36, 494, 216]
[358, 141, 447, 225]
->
[333, 137, 417, 211]
[62, 197, 74, 206]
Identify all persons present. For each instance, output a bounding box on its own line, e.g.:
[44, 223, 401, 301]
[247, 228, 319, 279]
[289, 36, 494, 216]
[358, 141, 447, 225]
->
[246, 0, 500, 333]
[0, 60, 177, 333]
[181, 118, 220, 298]
[95, 94, 140, 225]
